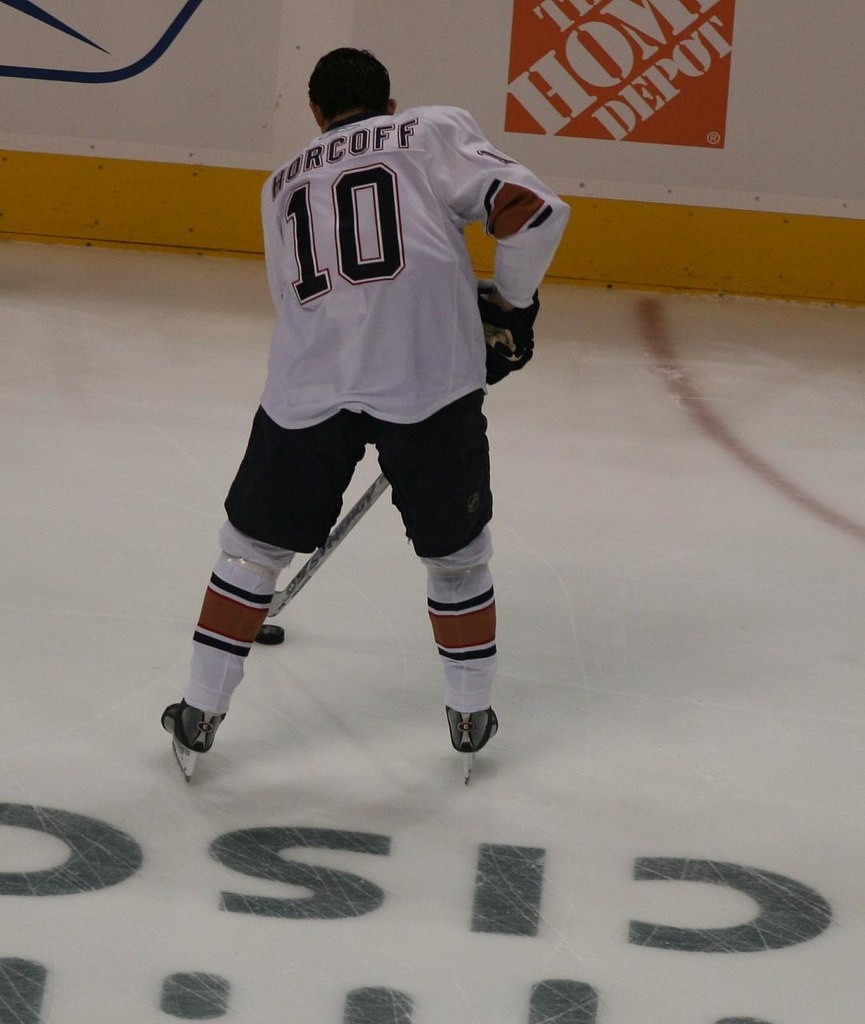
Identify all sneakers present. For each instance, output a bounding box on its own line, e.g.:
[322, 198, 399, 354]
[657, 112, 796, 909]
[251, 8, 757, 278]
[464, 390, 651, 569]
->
[445, 704, 498, 787]
[161, 695, 227, 782]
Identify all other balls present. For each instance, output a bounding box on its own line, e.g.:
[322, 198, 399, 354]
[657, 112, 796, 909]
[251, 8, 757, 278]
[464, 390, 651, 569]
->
[256, 624, 285, 645]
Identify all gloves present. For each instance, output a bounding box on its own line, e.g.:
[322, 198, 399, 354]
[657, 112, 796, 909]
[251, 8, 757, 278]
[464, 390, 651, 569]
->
[473, 277, 541, 386]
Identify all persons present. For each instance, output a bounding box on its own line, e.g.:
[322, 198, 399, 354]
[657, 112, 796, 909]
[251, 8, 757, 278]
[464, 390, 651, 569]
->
[160, 46, 572, 752]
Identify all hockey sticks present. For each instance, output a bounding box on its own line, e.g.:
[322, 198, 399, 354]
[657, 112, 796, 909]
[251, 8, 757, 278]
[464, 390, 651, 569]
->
[265, 324, 519, 622]
